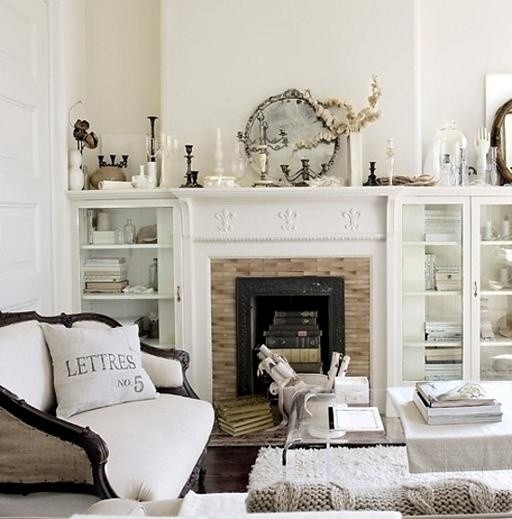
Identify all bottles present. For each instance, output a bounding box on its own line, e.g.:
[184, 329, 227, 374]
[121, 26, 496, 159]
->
[123, 219, 136, 244]
[150, 257, 158, 293]
[504, 217, 510, 236]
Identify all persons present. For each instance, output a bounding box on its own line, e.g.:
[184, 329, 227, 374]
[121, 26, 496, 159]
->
[473, 126, 492, 170]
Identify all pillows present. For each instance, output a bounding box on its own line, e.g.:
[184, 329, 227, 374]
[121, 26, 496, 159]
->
[38, 321, 160, 420]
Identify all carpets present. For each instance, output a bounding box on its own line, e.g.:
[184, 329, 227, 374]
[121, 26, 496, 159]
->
[247, 444, 512, 492]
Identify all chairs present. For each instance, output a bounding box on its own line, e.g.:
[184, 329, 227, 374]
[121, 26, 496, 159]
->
[0, 310, 215, 502]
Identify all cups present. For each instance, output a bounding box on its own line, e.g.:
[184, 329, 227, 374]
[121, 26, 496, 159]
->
[304, 392, 346, 439]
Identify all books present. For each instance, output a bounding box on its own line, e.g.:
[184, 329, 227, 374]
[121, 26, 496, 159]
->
[82, 257, 155, 293]
[252, 309, 323, 400]
[415, 400, 503, 425]
[211, 394, 276, 438]
[412, 380, 497, 407]
[424, 209, 495, 381]
[97, 180, 135, 190]
[89, 209, 100, 244]
[80, 208, 89, 245]
[414, 391, 502, 415]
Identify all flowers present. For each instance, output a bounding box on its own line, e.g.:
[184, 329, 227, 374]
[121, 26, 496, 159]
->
[67, 100, 100, 152]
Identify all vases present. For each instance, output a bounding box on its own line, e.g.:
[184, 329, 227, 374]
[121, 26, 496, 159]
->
[68, 148, 86, 191]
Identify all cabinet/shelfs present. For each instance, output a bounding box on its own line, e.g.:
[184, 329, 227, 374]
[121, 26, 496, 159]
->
[384, 190, 512, 420]
[70, 199, 186, 353]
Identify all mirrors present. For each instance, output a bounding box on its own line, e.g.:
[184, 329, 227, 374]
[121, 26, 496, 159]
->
[236, 89, 340, 185]
[492, 98, 512, 184]
